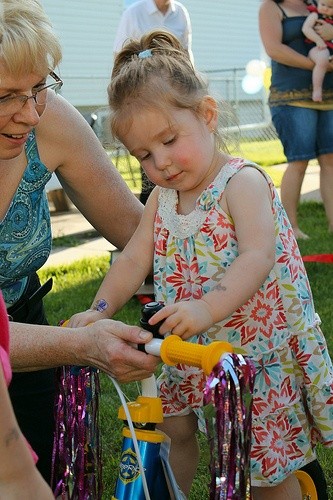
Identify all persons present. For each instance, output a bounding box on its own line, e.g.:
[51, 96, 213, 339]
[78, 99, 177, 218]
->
[257, 0, 333, 243]
[302, 1, 333, 103]
[0, 0, 159, 500]
[69, 31, 333, 499]
[117, 0, 192, 207]
[0, 290, 57, 500]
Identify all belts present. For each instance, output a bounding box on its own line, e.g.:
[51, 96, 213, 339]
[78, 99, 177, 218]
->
[7, 275, 53, 322]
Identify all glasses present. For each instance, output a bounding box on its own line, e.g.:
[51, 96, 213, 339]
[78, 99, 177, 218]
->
[0, 69, 63, 115]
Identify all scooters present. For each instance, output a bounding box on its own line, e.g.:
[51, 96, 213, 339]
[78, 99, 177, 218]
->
[58, 316, 328, 500]
